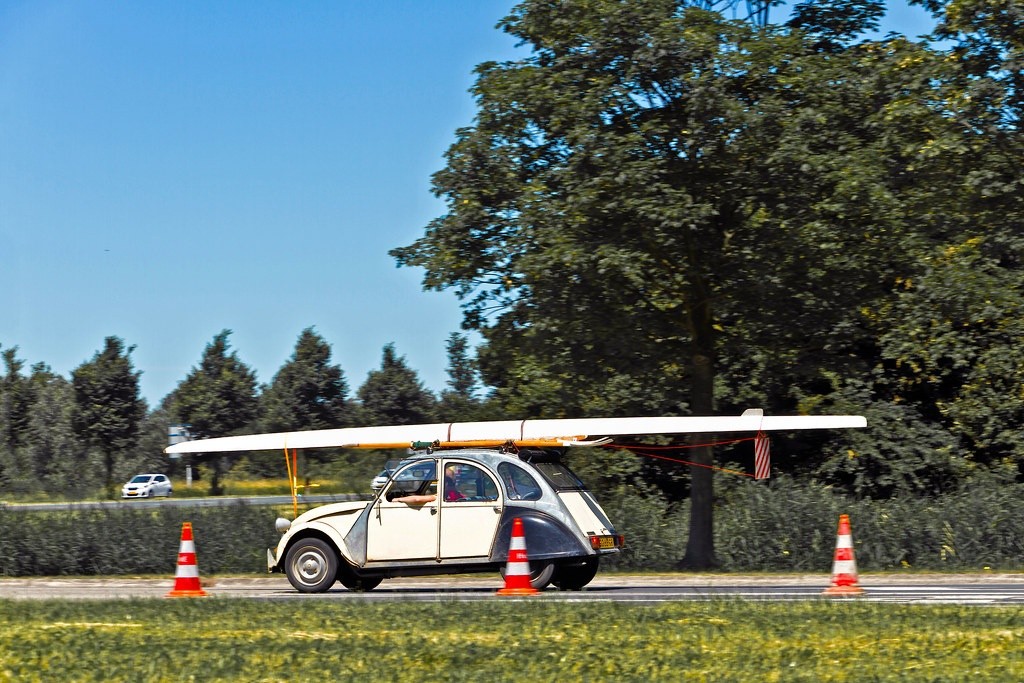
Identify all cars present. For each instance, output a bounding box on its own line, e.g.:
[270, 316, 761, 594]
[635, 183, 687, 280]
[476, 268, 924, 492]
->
[121, 474, 173, 499]
[269, 448, 624, 594]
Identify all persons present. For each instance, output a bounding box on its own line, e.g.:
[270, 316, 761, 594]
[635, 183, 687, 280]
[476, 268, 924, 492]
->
[391, 467, 466, 504]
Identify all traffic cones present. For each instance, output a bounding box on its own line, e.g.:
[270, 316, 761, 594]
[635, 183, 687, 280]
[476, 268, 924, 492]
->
[822, 515, 866, 595]
[497, 518, 540, 595]
[168, 522, 210, 596]
